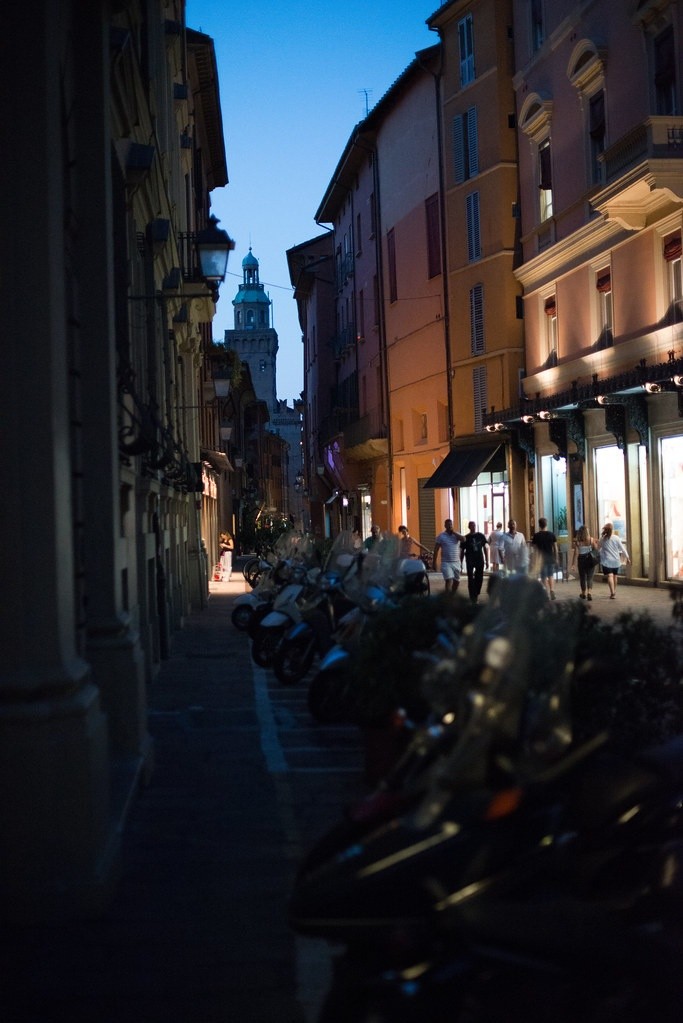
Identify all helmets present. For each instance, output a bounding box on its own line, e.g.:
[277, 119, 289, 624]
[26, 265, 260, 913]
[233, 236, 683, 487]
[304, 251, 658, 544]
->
[337, 554, 357, 577]
[399, 558, 426, 583]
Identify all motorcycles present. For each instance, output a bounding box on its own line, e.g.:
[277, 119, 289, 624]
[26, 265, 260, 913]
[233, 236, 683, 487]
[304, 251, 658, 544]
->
[229, 529, 683, 1023]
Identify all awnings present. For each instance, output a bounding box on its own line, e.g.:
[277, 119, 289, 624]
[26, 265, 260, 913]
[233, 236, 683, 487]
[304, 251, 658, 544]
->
[423, 441, 507, 488]
[203, 449, 234, 472]
[326, 494, 337, 503]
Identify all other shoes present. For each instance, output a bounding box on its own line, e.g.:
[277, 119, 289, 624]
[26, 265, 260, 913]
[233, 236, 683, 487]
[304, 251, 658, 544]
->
[610, 594, 616, 599]
[551, 591, 556, 600]
[588, 594, 592, 600]
[580, 594, 586, 598]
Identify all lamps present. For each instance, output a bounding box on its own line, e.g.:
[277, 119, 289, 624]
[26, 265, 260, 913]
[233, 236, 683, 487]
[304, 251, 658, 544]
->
[221, 416, 233, 439]
[177, 371, 234, 409]
[123, 217, 233, 301]
[236, 458, 242, 468]
[480, 376, 683, 432]
[292, 445, 326, 492]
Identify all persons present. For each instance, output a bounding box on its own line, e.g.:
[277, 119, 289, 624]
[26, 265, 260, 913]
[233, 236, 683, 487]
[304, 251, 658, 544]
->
[362, 525, 383, 552]
[218, 531, 234, 582]
[460, 522, 489, 604]
[488, 522, 502, 572]
[432, 519, 466, 593]
[596, 523, 630, 599]
[532, 518, 559, 600]
[240, 520, 322, 558]
[398, 525, 431, 556]
[351, 531, 362, 549]
[498, 520, 529, 574]
[571, 526, 599, 600]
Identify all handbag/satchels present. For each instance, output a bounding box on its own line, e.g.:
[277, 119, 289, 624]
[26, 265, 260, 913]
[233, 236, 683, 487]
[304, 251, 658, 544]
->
[589, 550, 600, 565]
